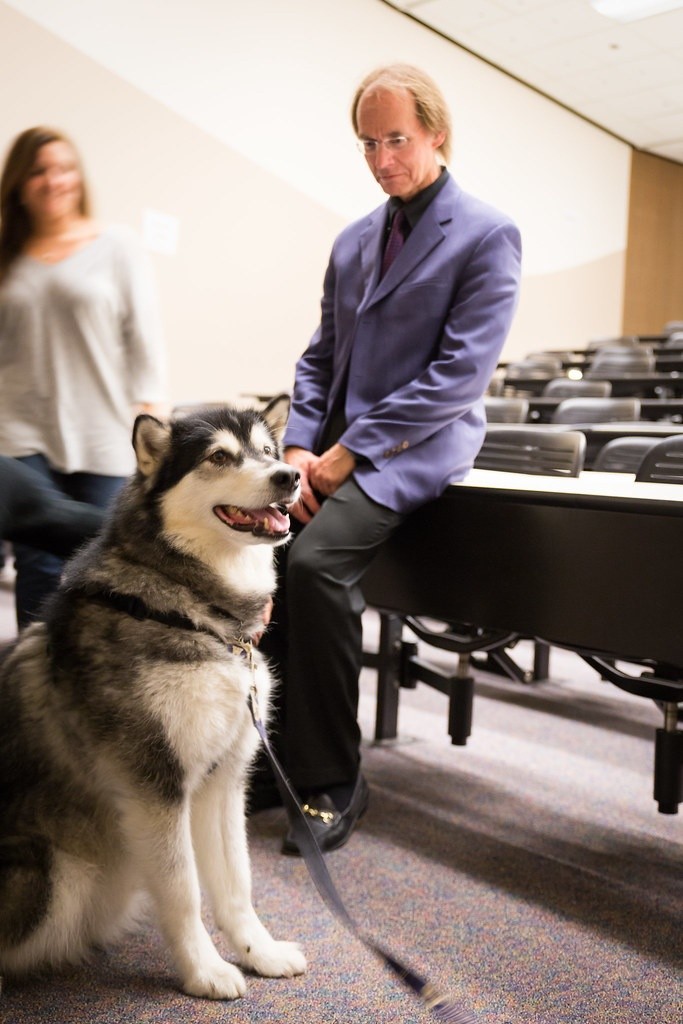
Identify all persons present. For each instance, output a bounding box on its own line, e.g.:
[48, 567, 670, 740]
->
[240, 64, 524, 859]
[0, 127, 168, 630]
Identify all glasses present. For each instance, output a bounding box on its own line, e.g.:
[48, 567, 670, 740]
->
[356, 136, 409, 153]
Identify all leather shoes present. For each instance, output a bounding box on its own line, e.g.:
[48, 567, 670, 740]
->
[283, 771, 368, 853]
[250, 772, 283, 809]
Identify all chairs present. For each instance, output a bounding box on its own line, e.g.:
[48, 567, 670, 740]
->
[579, 435, 683, 815]
[478, 320, 682, 473]
[395, 427, 587, 746]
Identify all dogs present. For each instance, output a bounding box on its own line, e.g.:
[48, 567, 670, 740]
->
[1, 394, 307, 1001]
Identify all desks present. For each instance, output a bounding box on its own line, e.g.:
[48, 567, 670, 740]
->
[280, 468, 682, 815]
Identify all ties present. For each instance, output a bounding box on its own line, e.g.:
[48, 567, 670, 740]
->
[383, 209, 404, 277]
